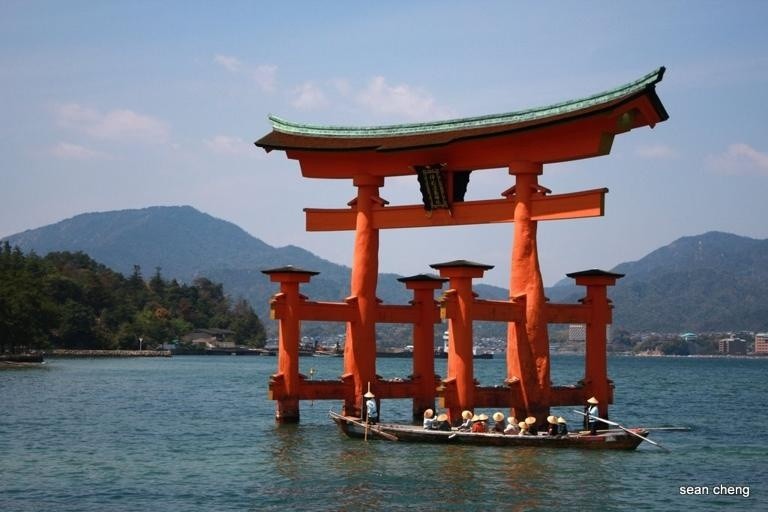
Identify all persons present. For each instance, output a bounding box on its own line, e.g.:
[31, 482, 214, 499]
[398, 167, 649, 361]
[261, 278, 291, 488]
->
[584, 403, 599, 435]
[423, 415, 438, 430]
[436, 421, 449, 431]
[451, 417, 567, 438]
[364, 397, 378, 425]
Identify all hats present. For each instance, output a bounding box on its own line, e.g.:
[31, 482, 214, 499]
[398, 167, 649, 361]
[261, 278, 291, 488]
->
[364, 392, 375, 398]
[518, 421, 529, 429]
[461, 409, 505, 423]
[507, 416, 518, 426]
[424, 409, 433, 418]
[437, 414, 448, 421]
[587, 397, 599, 404]
[547, 416, 566, 424]
[525, 416, 536, 424]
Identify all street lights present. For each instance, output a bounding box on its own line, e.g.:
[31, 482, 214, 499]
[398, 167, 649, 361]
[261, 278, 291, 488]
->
[138, 337, 144, 350]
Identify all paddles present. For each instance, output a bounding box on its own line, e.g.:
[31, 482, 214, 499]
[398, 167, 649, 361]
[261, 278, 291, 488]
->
[330, 410, 398, 442]
[573, 410, 663, 449]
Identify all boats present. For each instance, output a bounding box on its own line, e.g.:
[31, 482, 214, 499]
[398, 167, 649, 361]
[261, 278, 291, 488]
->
[328, 408, 648, 451]
[311, 348, 342, 359]
